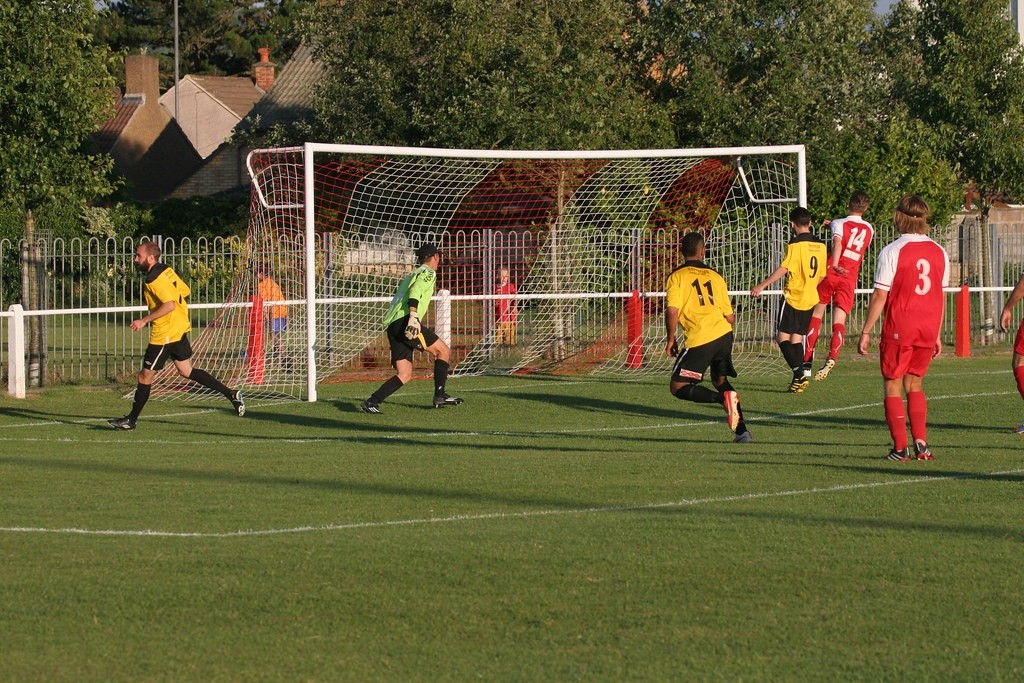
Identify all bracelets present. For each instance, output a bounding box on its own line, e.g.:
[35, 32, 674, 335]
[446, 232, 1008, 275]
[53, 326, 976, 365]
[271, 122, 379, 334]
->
[861, 331, 870, 334]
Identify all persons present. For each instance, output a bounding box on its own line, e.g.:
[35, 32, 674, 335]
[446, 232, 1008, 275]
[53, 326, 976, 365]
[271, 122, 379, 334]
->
[107, 241, 246, 430]
[361, 244, 464, 413]
[857, 194, 949, 460]
[666, 231, 752, 442]
[802, 190, 874, 380]
[238, 266, 290, 371]
[750, 206, 827, 396]
[1000, 267, 1024, 433]
[492, 265, 517, 346]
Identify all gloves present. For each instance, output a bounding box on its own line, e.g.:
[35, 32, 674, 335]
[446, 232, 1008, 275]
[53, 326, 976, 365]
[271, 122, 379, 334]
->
[404, 312, 422, 340]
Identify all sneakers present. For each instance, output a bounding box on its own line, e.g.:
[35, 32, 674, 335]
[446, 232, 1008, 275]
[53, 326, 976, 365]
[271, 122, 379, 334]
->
[230, 389, 245, 417]
[912, 443, 933, 461]
[815, 358, 835, 383]
[722, 391, 741, 432]
[732, 431, 752, 444]
[885, 447, 912, 462]
[107, 416, 137, 431]
[362, 399, 383, 414]
[432, 393, 464, 408]
[787, 377, 809, 394]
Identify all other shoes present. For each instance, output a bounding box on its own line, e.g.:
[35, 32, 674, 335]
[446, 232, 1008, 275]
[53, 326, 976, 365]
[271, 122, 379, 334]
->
[1016, 422, 1024, 434]
